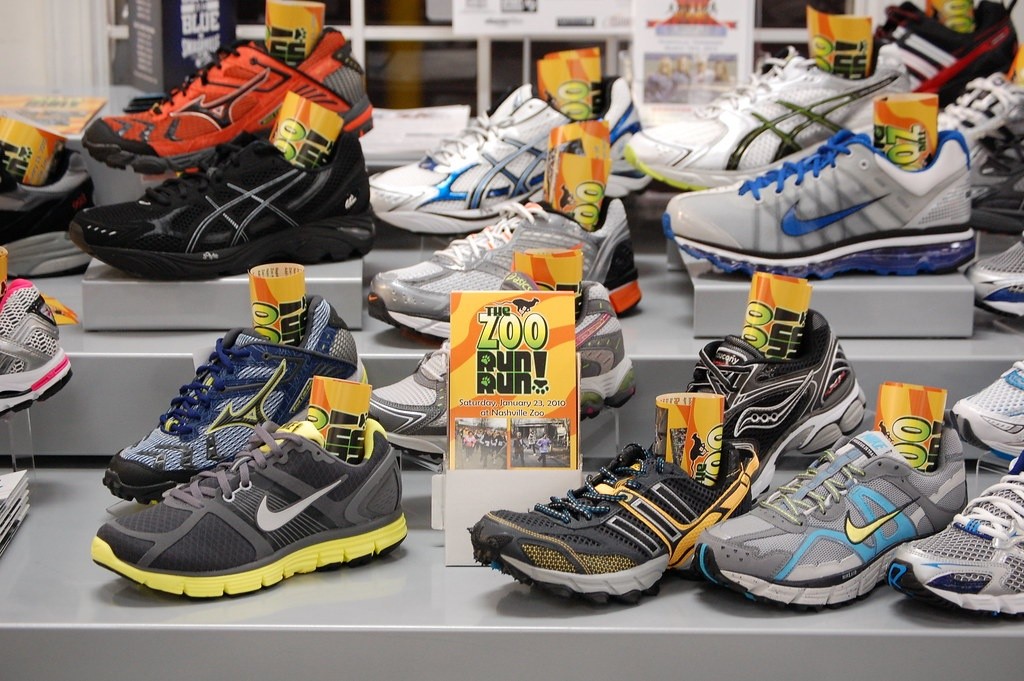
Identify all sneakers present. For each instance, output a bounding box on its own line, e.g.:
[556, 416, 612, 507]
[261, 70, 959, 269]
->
[370, 272, 636, 460]
[82, 27, 374, 174]
[884, 449, 1024, 621]
[689, 410, 968, 611]
[0, 149, 96, 279]
[102, 294, 370, 505]
[937, 71, 1024, 185]
[0, 279, 73, 418]
[684, 308, 866, 505]
[368, 195, 642, 343]
[952, 359, 1024, 468]
[965, 233, 1024, 319]
[874, 0, 1019, 106]
[368, 77, 653, 236]
[971, 164, 1024, 234]
[662, 128, 976, 280]
[68, 131, 375, 280]
[467, 440, 752, 605]
[93, 419, 407, 597]
[623, 38, 910, 191]
